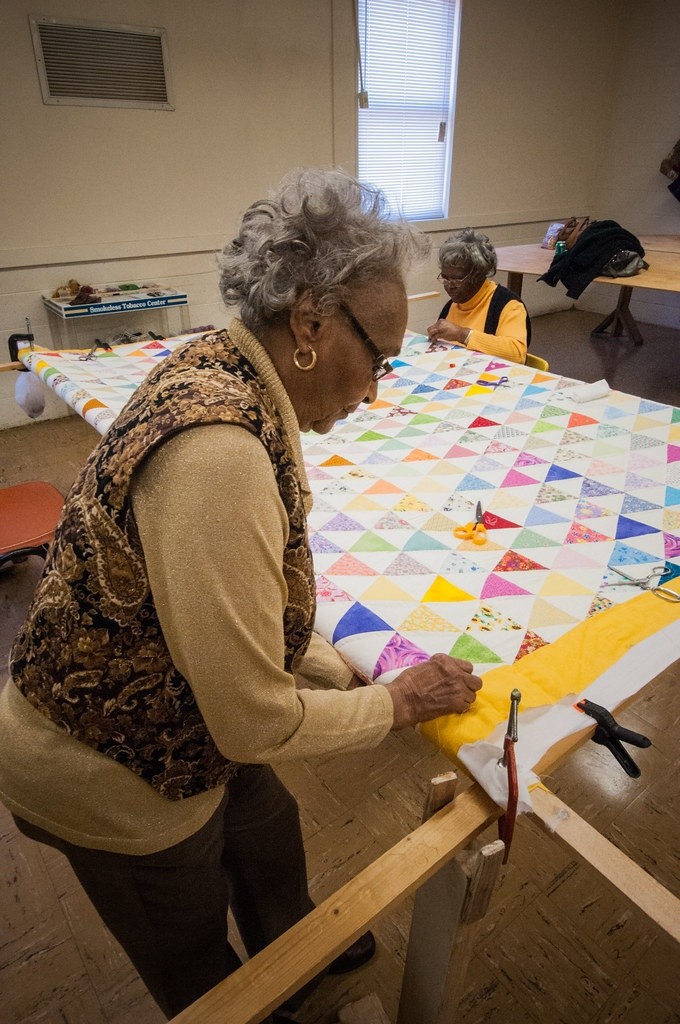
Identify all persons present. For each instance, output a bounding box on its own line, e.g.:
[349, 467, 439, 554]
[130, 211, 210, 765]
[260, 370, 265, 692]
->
[424, 229, 532, 365]
[1, 167, 482, 1024]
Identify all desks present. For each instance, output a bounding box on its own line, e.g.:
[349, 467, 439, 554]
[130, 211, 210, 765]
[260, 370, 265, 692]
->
[494, 234, 680, 346]
[40, 281, 191, 416]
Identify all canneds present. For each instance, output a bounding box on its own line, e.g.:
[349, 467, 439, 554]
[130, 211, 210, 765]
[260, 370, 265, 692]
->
[555, 241, 567, 255]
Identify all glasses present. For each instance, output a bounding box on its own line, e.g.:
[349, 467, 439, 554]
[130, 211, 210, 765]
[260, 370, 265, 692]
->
[338, 299, 393, 382]
[437, 266, 475, 289]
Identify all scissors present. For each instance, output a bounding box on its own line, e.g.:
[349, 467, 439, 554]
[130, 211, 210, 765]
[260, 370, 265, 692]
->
[79, 351, 98, 362]
[454, 501, 487, 545]
[477, 376, 512, 391]
[146, 291, 162, 297]
[600, 563, 680, 606]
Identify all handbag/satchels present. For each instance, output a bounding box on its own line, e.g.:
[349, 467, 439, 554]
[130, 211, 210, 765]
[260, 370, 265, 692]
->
[541, 223, 565, 250]
[601, 248, 650, 278]
[558, 216, 590, 250]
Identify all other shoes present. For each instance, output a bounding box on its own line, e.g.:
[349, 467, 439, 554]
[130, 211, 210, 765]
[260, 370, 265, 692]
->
[326, 929, 376, 974]
